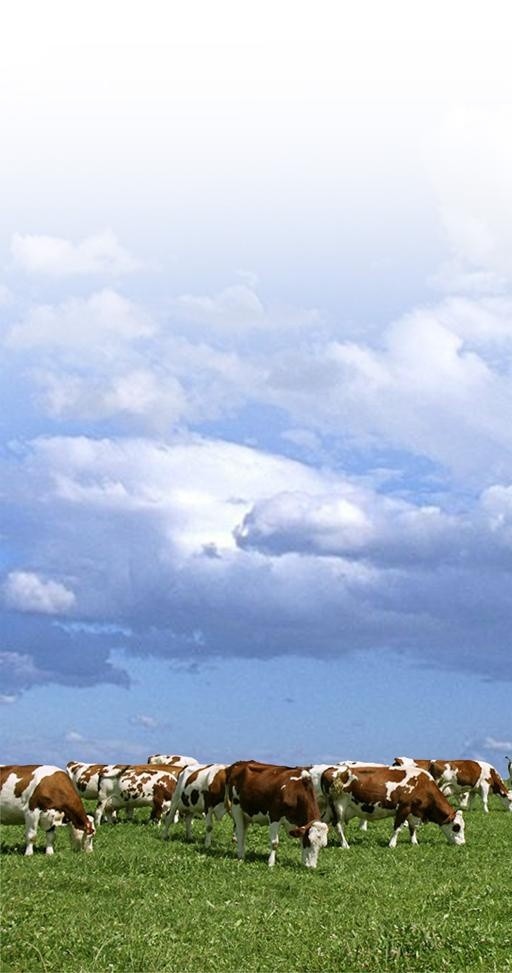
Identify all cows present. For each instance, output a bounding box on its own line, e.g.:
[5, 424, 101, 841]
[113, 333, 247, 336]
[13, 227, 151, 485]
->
[0, 765, 96, 857]
[225, 760, 330, 868]
[303, 756, 512, 849]
[66, 752, 237, 852]
